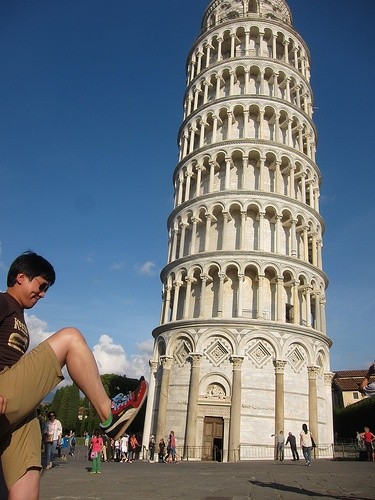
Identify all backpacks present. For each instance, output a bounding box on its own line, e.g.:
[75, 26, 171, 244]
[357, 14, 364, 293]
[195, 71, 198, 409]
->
[72, 439, 76, 447]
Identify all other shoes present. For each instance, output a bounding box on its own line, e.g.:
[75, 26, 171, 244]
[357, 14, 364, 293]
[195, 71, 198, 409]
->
[146, 459, 154, 463]
[306, 461, 311, 466]
[100, 458, 135, 464]
[158, 458, 178, 463]
[292, 458, 299, 461]
[90, 471, 102, 474]
[46, 465, 50, 470]
[276, 459, 284, 462]
[49, 462, 52, 469]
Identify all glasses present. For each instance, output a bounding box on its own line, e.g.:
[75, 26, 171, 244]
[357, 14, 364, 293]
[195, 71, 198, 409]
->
[49, 415, 55, 417]
[33, 277, 49, 292]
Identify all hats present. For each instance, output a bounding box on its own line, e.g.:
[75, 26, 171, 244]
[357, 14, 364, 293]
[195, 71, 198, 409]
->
[150, 434, 155, 439]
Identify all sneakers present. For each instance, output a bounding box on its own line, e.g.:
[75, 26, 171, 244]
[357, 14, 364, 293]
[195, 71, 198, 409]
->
[99, 380, 148, 440]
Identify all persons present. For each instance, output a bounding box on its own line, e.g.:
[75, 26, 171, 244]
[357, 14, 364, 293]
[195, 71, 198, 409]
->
[300, 424, 313, 466]
[149, 435, 156, 461]
[360, 426, 375, 461]
[275, 431, 285, 460]
[0, 251, 148, 500]
[359, 363, 375, 395]
[90, 430, 103, 474]
[36, 409, 62, 470]
[164, 431, 176, 463]
[356, 431, 361, 448]
[83, 430, 141, 464]
[58, 431, 77, 461]
[159, 438, 165, 463]
[285, 432, 299, 461]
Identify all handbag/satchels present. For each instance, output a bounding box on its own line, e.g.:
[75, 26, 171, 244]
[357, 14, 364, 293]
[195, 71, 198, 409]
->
[91, 452, 97, 459]
[311, 437, 316, 448]
[45, 434, 54, 443]
